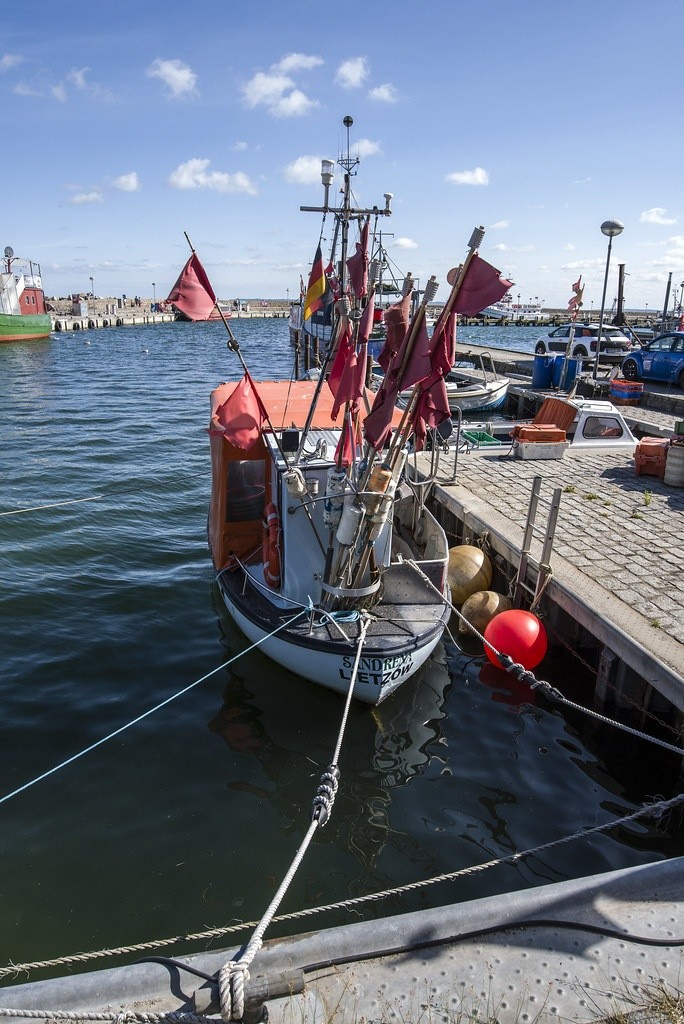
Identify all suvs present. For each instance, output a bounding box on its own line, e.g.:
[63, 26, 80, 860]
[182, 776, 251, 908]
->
[535, 323, 629, 369]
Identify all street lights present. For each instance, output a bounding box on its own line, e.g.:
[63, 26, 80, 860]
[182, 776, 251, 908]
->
[152, 283, 155, 314]
[593, 221, 625, 380]
[89, 277, 95, 315]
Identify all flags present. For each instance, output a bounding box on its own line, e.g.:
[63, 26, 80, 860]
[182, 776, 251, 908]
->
[295, 242, 519, 467]
[567, 275, 581, 320]
[211, 372, 266, 458]
[164, 255, 217, 322]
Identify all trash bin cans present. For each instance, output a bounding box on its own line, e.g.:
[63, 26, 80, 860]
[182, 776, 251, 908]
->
[533, 354, 554, 389]
[552, 355, 578, 393]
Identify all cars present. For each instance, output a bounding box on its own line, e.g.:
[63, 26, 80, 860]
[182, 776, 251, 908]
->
[621, 332, 684, 388]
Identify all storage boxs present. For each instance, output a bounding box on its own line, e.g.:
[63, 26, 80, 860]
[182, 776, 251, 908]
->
[461, 430, 500, 446]
[636, 436, 672, 479]
[608, 378, 644, 405]
[513, 424, 566, 442]
[512, 439, 569, 459]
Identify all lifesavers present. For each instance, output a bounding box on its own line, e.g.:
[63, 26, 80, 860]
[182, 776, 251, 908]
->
[262, 502, 280, 588]
[117, 319, 121, 326]
[89, 320, 95, 328]
[56, 321, 61, 331]
[73, 323, 79, 330]
[103, 320, 108, 327]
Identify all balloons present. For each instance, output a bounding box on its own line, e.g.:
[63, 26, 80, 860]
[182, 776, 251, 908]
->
[483, 609, 548, 673]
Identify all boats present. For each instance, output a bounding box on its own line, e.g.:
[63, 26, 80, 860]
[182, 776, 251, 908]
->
[385, 398, 639, 457]
[0, 246, 52, 342]
[475, 293, 550, 321]
[206, 115, 452, 710]
[288, 273, 388, 362]
[374, 351, 509, 414]
[191, 298, 231, 322]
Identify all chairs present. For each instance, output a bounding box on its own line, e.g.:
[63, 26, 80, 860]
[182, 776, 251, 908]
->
[590, 364, 621, 400]
